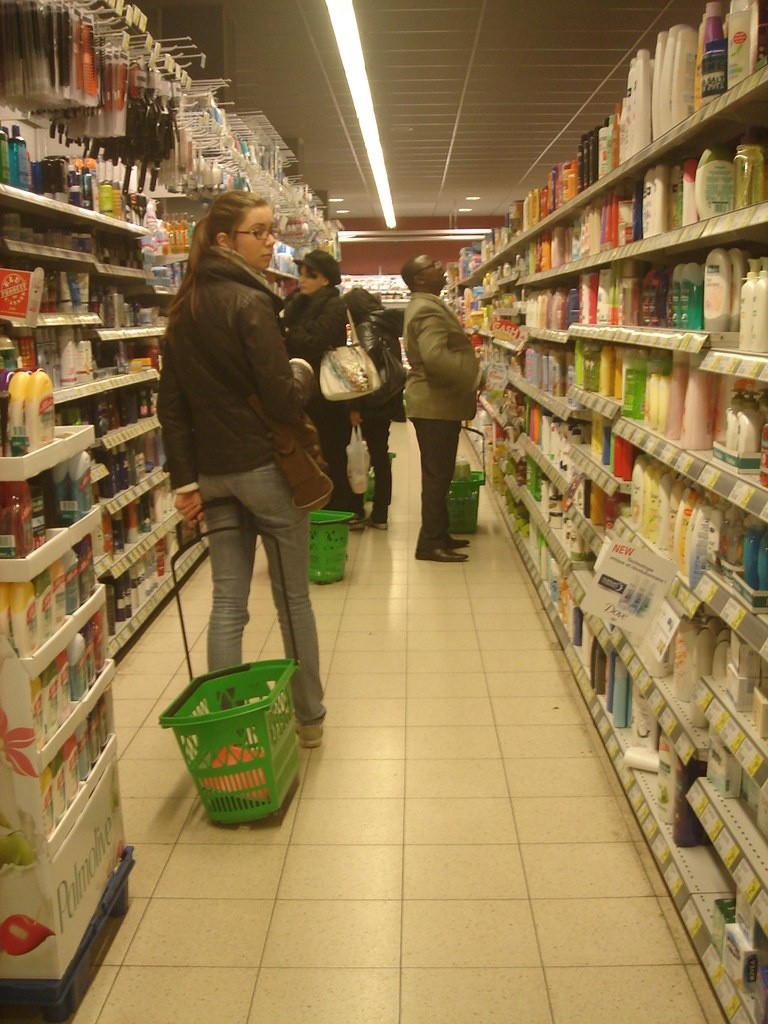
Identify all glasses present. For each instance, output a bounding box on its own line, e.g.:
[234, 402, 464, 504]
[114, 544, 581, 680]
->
[235, 223, 281, 241]
[411, 260, 442, 283]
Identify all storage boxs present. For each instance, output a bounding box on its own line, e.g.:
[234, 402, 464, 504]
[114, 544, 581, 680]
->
[706, 630, 767, 1024]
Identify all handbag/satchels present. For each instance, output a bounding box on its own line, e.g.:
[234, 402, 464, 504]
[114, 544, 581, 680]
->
[345, 423, 371, 494]
[345, 322, 405, 421]
[267, 407, 334, 512]
[319, 304, 382, 401]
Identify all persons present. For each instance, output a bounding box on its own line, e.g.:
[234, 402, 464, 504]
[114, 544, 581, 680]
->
[401, 253, 485, 562]
[280, 250, 353, 514]
[342, 287, 406, 530]
[155, 190, 327, 748]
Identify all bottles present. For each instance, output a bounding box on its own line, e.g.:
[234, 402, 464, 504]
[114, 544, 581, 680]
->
[728, 248, 745, 332]
[727, 0, 751, 91]
[740, 257, 768, 354]
[520, 0, 731, 847]
[732, 133, 764, 211]
[0, 122, 188, 836]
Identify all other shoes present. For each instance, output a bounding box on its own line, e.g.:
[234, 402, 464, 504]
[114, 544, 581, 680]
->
[346, 513, 366, 529]
[292, 711, 323, 747]
[365, 517, 388, 530]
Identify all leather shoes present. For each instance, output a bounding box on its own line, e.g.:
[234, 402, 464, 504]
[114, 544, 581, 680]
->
[448, 537, 469, 548]
[416, 544, 469, 562]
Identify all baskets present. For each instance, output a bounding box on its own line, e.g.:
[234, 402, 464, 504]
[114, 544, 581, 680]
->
[158, 497, 300, 825]
[445, 426, 484, 535]
[366, 450, 396, 502]
[306, 509, 356, 584]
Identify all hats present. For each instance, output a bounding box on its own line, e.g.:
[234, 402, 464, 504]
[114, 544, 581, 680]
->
[293, 249, 342, 285]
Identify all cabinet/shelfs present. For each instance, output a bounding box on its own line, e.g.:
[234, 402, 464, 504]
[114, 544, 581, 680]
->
[382, 275, 473, 445]
[0, 184, 300, 1024]
[474, 68, 768, 1024]
